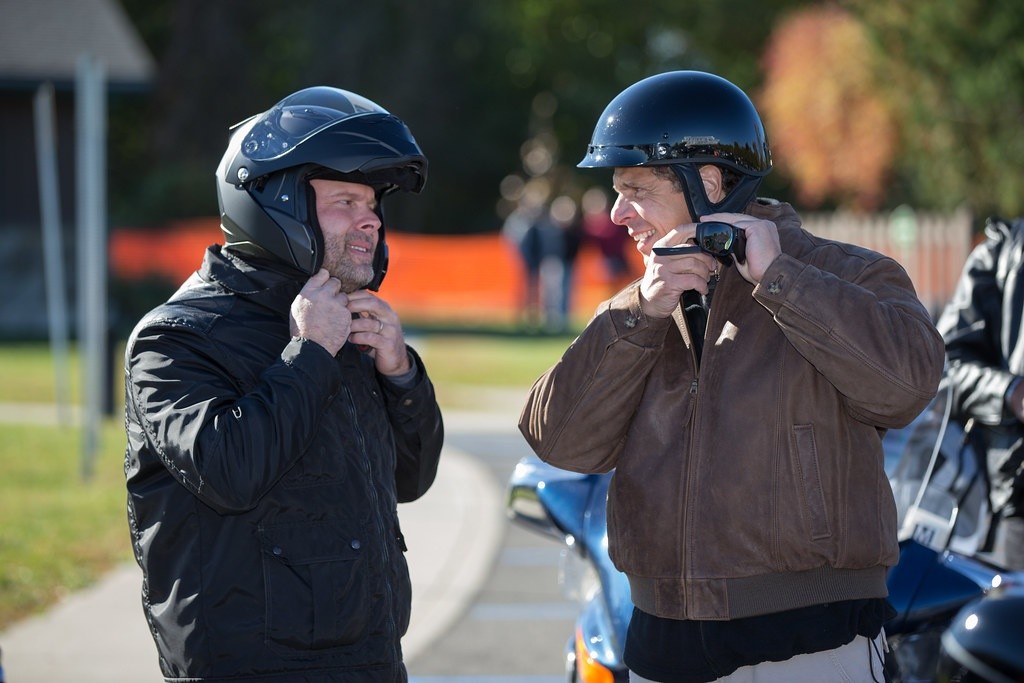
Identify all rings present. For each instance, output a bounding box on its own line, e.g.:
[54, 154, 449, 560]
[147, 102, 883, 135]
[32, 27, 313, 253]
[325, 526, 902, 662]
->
[376, 319, 383, 334]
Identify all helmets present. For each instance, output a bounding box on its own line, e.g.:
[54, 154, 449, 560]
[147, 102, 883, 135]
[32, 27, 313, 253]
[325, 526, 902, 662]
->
[576, 69, 774, 178]
[217, 86, 430, 291]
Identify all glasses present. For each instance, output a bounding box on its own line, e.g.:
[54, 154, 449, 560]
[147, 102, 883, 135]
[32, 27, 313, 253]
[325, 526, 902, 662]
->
[652, 220, 748, 268]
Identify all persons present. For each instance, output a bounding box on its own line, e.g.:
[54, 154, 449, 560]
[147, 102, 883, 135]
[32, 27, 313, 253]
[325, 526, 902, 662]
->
[119, 87, 451, 683]
[936, 216, 1024, 570]
[504, 208, 633, 336]
[519, 70, 945, 683]
